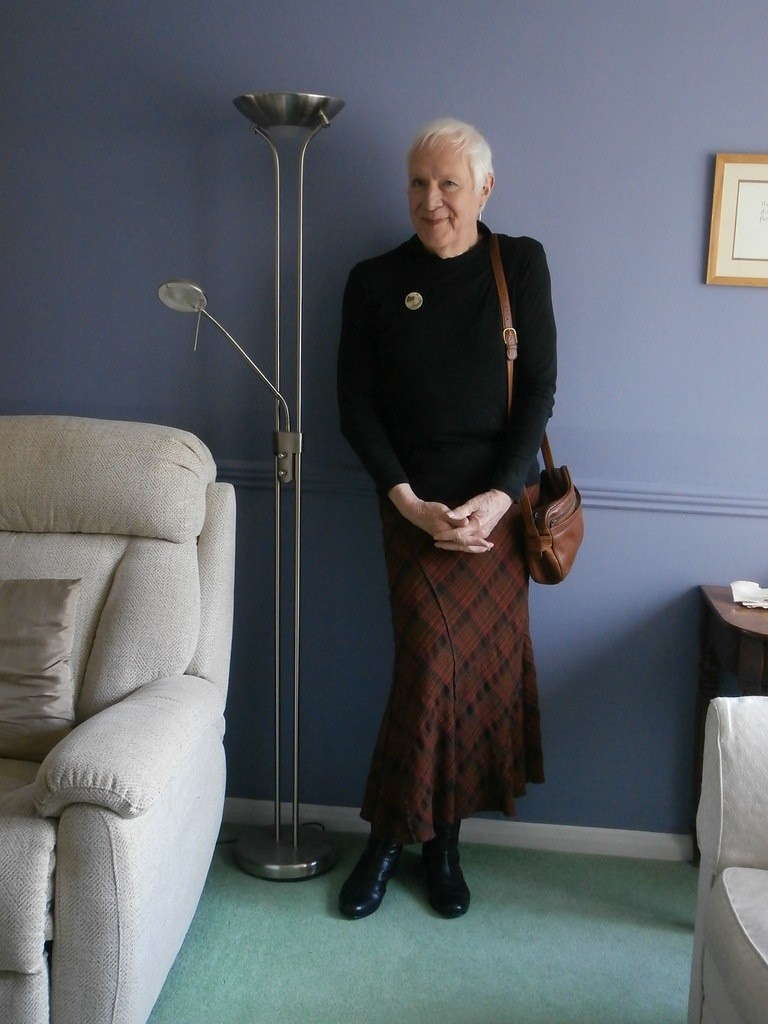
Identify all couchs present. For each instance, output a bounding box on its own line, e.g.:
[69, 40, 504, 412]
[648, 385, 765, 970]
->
[689, 696, 768, 1024]
[0, 412, 237, 1024]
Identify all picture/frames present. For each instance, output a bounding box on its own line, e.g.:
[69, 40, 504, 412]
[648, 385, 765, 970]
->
[705, 153, 768, 287]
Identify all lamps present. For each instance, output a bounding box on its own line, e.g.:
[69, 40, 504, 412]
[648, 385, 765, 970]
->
[158, 92, 345, 882]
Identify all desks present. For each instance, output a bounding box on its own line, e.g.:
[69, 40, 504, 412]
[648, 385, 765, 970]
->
[692, 584, 768, 868]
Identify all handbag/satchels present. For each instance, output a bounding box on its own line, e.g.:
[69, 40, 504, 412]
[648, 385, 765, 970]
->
[525, 466, 584, 585]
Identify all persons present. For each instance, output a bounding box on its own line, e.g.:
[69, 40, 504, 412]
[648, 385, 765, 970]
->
[336, 119, 558, 920]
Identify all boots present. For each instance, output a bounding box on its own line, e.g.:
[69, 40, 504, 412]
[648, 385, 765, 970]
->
[422, 818, 470, 917]
[339, 823, 406, 918]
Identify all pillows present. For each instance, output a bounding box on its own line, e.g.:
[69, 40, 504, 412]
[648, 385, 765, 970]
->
[0, 579, 84, 763]
